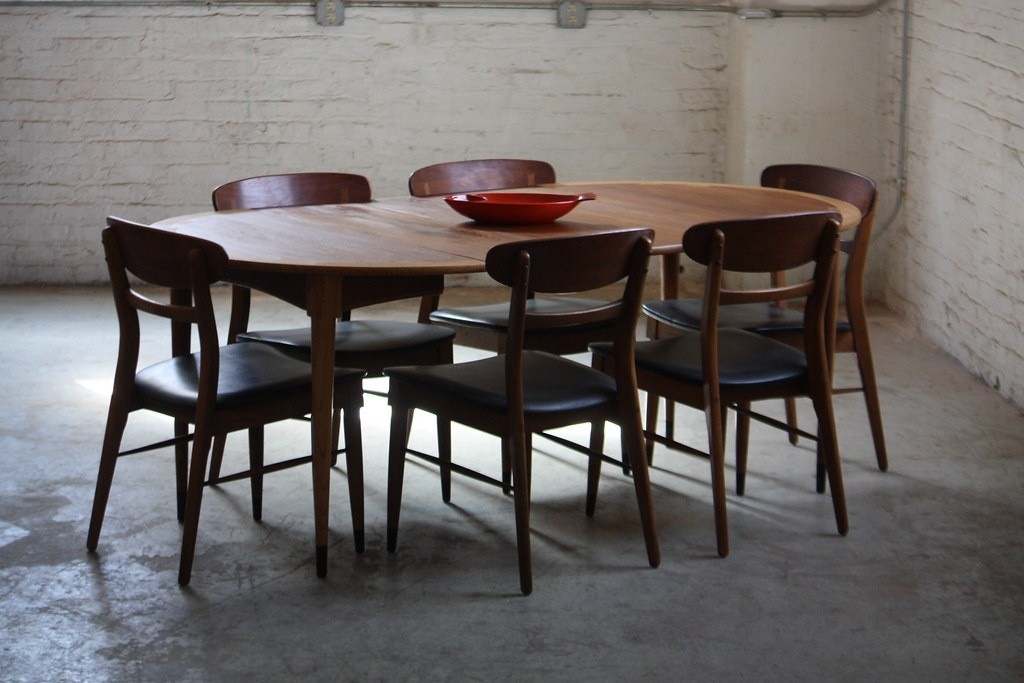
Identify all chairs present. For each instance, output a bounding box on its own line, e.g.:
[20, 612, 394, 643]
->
[84, 214, 368, 590]
[409, 159, 631, 495]
[643, 163, 889, 473]
[208, 171, 455, 526]
[648, 211, 851, 558]
[382, 229, 662, 599]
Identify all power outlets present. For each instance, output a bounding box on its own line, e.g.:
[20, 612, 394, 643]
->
[558, 1, 586, 28]
[317, 0, 345, 26]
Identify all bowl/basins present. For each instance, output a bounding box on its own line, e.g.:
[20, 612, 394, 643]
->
[442, 192, 596, 223]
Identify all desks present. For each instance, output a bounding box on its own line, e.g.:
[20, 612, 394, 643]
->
[147, 181, 861, 553]
